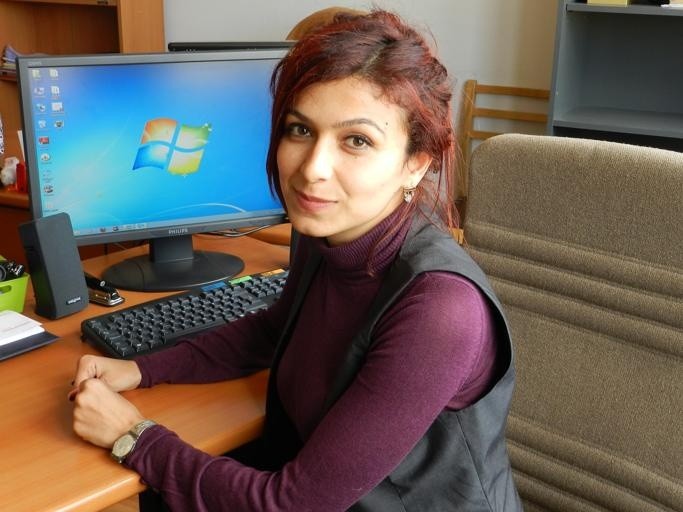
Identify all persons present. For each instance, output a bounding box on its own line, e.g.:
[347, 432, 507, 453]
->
[63, 3, 529, 512]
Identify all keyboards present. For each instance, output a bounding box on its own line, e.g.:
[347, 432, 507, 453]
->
[80, 264, 288, 360]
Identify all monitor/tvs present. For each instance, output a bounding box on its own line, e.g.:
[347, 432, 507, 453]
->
[14, 47, 290, 293]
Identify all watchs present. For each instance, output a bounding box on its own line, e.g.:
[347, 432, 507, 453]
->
[110, 419, 156, 463]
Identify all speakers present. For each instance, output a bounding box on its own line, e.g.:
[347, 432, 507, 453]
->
[18, 212, 90, 320]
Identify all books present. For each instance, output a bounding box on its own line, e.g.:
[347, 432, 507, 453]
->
[2, 43, 53, 73]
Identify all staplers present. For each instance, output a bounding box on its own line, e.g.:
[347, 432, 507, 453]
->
[83, 271, 125, 306]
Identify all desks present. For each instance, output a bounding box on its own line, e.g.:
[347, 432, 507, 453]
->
[2, 234, 289, 510]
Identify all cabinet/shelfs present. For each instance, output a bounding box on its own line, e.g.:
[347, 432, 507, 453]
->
[546, 0, 682, 152]
